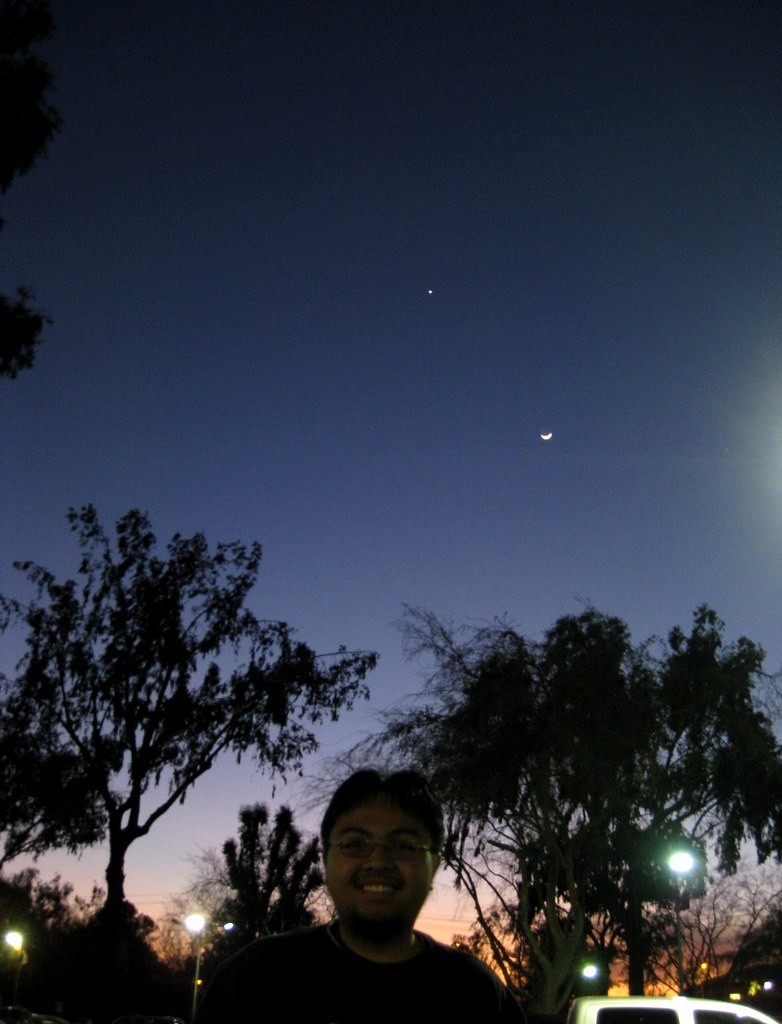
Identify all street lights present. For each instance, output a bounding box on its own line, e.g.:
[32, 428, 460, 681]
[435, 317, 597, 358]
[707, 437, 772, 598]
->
[6, 929, 30, 1003]
[184, 910, 206, 1024]
[667, 849, 695, 993]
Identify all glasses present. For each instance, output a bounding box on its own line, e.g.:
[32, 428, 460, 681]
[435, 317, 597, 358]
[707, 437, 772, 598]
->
[328, 833, 434, 860]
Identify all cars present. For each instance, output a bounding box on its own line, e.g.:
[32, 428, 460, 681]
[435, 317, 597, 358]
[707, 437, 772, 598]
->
[0, 1002, 187, 1024]
[567, 992, 782, 1024]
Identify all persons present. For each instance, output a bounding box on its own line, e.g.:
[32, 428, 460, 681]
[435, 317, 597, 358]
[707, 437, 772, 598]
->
[191, 769, 528, 1024]
